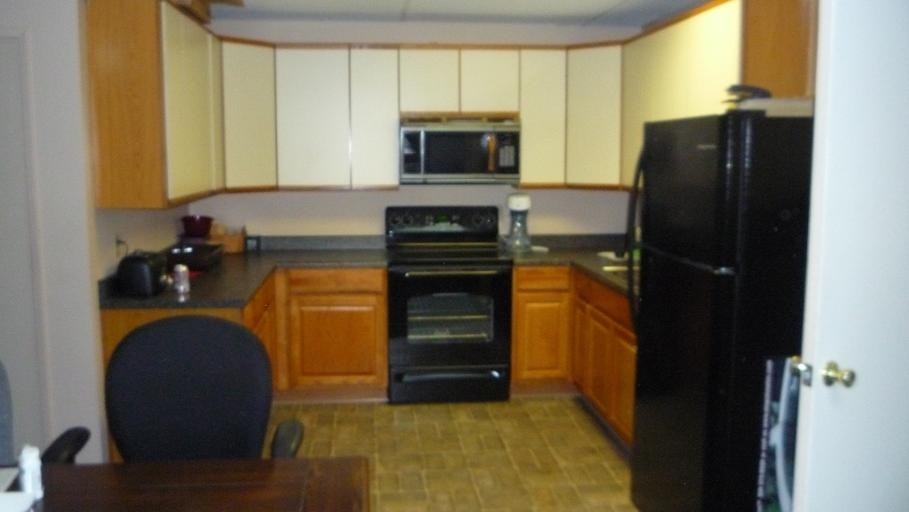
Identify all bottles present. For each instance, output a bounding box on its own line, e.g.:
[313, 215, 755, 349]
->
[15, 445, 43, 497]
[170, 257, 191, 294]
[506, 192, 531, 254]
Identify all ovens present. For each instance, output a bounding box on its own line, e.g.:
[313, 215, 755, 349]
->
[387, 268, 511, 405]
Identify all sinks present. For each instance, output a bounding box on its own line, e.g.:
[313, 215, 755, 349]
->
[601, 265, 639, 292]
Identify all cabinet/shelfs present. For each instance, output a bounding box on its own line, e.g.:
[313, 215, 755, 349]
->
[623, 3, 814, 189]
[566, 39, 625, 190]
[520, 46, 566, 188]
[276, 43, 350, 190]
[99, 271, 276, 464]
[400, 44, 519, 116]
[573, 264, 639, 447]
[225, 36, 276, 191]
[511, 266, 575, 396]
[349, 43, 401, 190]
[276, 269, 388, 400]
[208, 31, 225, 195]
[81, 0, 210, 209]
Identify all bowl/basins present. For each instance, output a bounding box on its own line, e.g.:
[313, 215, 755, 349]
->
[181, 215, 213, 236]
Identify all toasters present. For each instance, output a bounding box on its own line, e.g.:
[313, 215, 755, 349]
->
[121, 249, 166, 293]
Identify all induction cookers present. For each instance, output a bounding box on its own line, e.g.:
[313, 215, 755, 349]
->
[387, 243, 512, 264]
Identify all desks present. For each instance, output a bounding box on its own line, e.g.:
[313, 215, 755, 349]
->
[0, 455, 371, 512]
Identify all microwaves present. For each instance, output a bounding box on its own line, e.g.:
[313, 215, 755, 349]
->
[397, 121, 520, 185]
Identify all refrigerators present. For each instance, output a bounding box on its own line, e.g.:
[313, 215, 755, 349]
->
[625, 110, 813, 512]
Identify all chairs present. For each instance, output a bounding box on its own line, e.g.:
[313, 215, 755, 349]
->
[42, 313, 304, 464]
